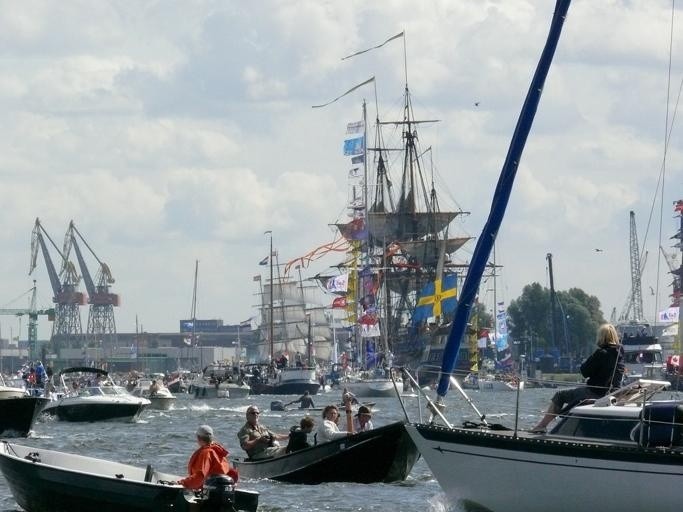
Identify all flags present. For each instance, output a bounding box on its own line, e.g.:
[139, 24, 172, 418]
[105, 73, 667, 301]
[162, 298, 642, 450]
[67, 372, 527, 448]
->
[477, 300, 513, 369]
[253, 249, 301, 282]
[655, 191, 683, 376]
[406, 270, 462, 325]
[323, 115, 404, 377]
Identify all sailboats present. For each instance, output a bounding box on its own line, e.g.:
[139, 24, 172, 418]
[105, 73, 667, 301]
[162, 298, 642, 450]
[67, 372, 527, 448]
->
[247, 231, 321, 396]
[309, 307, 334, 382]
[388, 1, 682, 512]
[311, 33, 502, 390]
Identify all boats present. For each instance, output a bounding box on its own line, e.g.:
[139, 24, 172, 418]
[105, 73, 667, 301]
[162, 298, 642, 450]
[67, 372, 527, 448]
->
[43, 367, 151, 422]
[231, 421, 424, 483]
[0, 437, 259, 512]
[0, 369, 51, 436]
[342, 375, 405, 398]
[478, 377, 526, 393]
[612, 322, 665, 382]
[131, 380, 178, 411]
[0, 359, 250, 398]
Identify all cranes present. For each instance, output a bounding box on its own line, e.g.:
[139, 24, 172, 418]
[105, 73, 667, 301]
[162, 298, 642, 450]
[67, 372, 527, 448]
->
[0, 280, 55, 362]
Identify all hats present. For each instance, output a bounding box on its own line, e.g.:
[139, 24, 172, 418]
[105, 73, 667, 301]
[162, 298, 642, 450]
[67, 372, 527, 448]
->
[198, 426, 214, 438]
[355, 406, 370, 416]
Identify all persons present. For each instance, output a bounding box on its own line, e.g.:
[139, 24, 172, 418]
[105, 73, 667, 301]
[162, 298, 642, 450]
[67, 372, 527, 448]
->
[342, 405, 373, 434]
[165, 422, 240, 490]
[341, 387, 359, 404]
[1, 344, 319, 412]
[235, 404, 289, 461]
[333, 411, 341, 427]
[524, 320, 627, 435]
[283, 416, 314, 454]
[313, 405, 353, 446]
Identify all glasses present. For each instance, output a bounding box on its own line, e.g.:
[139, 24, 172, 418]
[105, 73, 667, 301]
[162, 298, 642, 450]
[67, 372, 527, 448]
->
[250, 412, 259, 415]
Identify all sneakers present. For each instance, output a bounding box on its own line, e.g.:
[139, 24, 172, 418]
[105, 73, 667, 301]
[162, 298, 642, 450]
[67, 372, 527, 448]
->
[528, 427, 547, 433]
